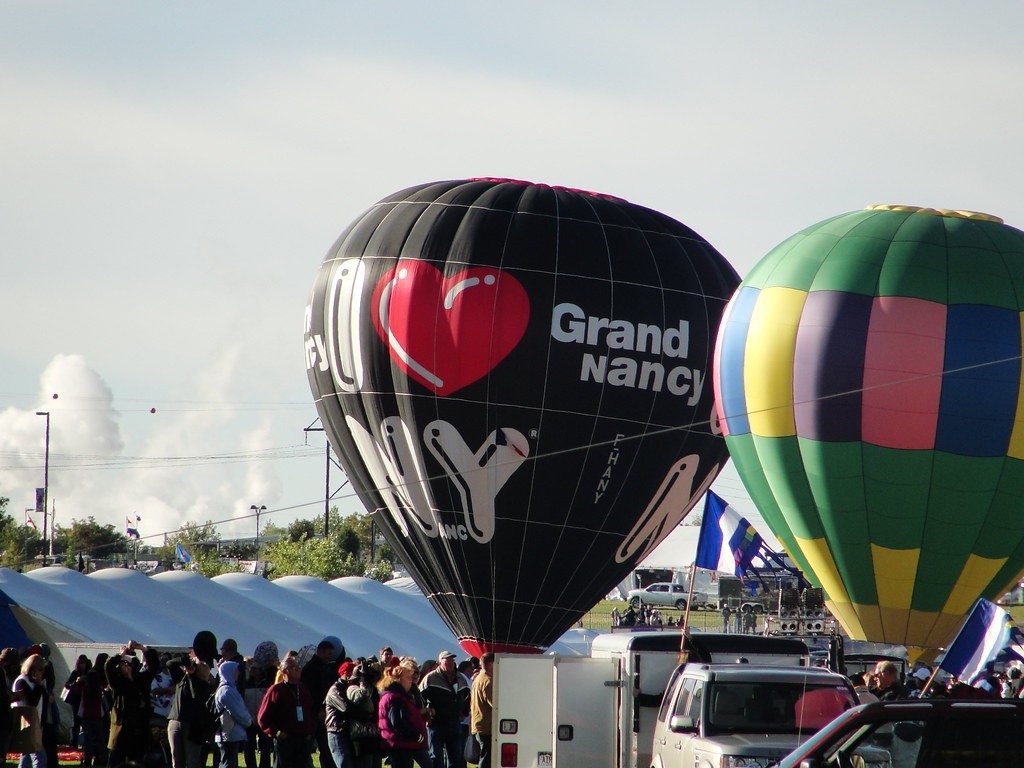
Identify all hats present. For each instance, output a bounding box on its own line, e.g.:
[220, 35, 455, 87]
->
[380, 646, 395, 658]
[368, 654, 379, 664]
[188, 631, 217, 649]
[296, 645, 318, 670]
[339, 662, 357, 675]
[439, 650, 457, 660]
[253, 640, 282, 669]
[220, 639, 238, 650]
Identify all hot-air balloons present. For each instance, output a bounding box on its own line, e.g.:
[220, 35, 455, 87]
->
[149, 407, 157, 414]
[52, 392, 58, 399]
[711, 204, 1024, 700]
[303, 176, 747, 673]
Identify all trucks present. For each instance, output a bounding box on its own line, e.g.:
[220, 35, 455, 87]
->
[493, 632, 862, 768]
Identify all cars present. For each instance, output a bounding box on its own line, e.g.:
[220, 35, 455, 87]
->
[761, 698, 1024, 768]
[628, 580, 711, 612]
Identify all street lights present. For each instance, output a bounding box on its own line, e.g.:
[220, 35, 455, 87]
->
[34, 411, 50, 568]
[250, 504, 266, 561]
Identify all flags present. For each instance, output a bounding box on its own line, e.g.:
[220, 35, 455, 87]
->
[127, 515, 142, 525]
[177, 542, 191, 563]
[26, 514, 37, 530]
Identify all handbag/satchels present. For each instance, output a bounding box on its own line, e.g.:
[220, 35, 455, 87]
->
[349, 718, 379, 740]
[60, 685, 70, 701]
[10, 703, 45, 752]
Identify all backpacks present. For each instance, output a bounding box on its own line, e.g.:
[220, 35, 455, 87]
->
[100, 686, 116, 711]
[204, 692, 226, 725]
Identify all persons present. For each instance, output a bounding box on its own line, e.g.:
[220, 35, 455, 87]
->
[0, 631, 494, 768]
[612, 603, 684, 627]
[849, 661, 1024, 705]
[722, 603, 757, 634]
